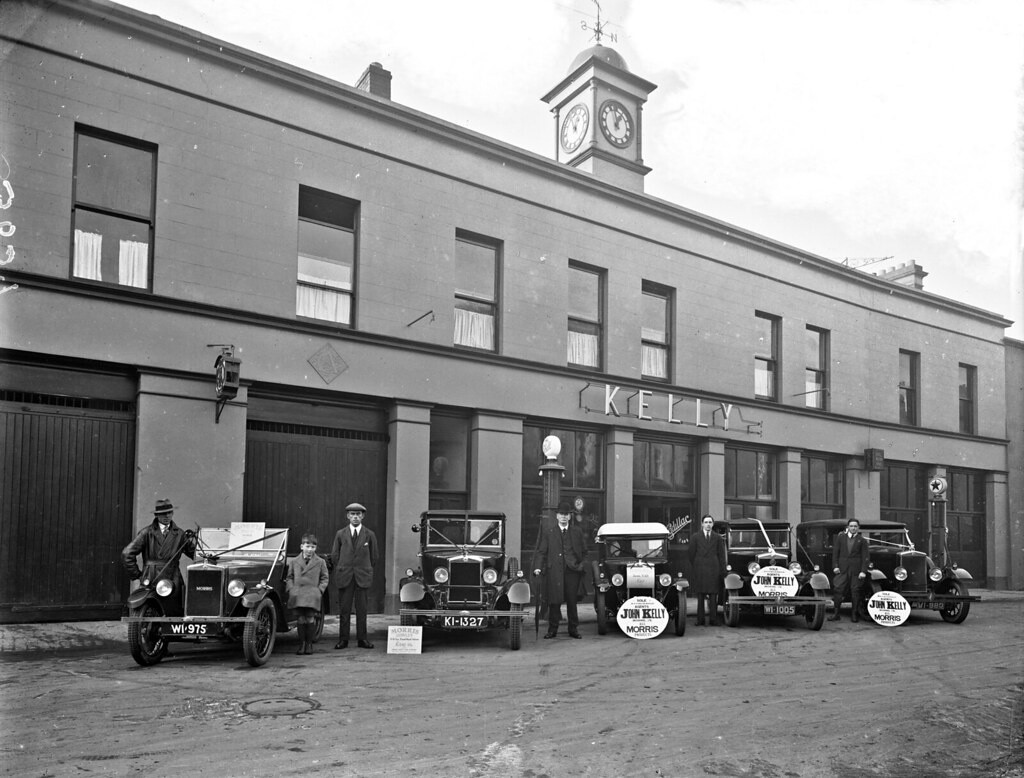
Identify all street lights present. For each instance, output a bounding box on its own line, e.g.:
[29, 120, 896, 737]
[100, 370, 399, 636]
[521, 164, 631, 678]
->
[537, 433, 564, 538]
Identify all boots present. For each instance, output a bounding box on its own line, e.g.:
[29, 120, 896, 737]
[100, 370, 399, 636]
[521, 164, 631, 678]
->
[304, 624, 313, 654]
[297, 623, 306, 655]
[852, 609, 858, 622]
[827, 608, 840, 620]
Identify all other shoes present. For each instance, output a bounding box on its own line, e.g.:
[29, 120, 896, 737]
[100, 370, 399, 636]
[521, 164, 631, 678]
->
[695, 621, 705, 626]
[570, 632, 581, 638]
[710, 621, 720, 626]
[545, 632, 556, 639]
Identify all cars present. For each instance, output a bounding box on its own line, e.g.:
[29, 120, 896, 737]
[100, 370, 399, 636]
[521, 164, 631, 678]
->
[397, 509, 531, 651]
[592, 522, 689, 637]
[120, 522, 324, 666]
[792, 519, 983, 624]
[712, 516, 835, 630]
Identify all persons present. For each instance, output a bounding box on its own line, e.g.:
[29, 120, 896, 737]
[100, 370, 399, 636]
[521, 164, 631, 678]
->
[827, 519, 867, 622]
[122, 499, 195, 583]
[286, 533, 329, 654]
[534, 501, 586, 639]
[331, 503, 377, 650]
[685, 514, 726, 625]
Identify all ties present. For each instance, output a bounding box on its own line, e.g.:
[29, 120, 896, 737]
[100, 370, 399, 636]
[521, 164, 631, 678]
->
[305, 558, 309, 564]
[563, 528, 567, 535]
[163, 525, 169, 538]
[352, 529, 357, 543]
[706, 531, 709, 541]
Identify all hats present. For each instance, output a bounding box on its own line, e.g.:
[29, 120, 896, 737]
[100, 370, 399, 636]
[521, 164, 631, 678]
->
[554, 502, 574, 514]
[345, 503, 366, 512]
[150, 499, 178, 514]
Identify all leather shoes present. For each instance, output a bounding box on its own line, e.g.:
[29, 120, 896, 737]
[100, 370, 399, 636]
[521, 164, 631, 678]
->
[358, 639, 374, 648]
[335, 640, 347, 649]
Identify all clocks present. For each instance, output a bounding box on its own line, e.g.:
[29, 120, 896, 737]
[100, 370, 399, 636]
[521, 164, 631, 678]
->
[560, 103, 589, 153]
[598, 99, 635, 149]
